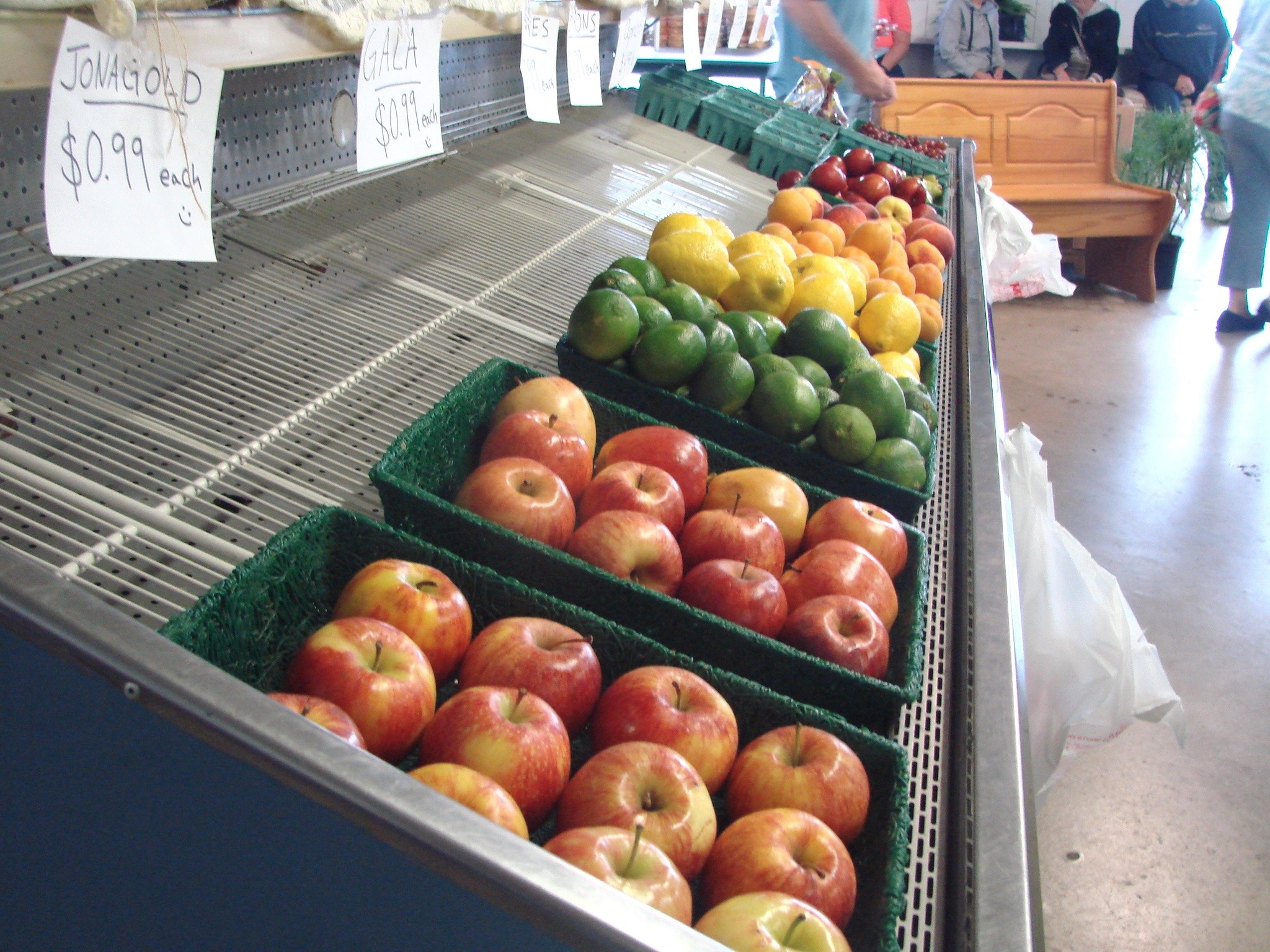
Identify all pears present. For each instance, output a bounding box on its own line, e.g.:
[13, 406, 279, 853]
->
[901, 170, 942, 197]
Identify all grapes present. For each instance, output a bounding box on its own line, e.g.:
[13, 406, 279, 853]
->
[856, 120, 947, 162]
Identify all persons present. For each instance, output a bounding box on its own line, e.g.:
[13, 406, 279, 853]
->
[1131, 0, 1232, 222]
[1040, 1, 1121, 97]
[932, 0, 1018, 81]
[1210, 0, 1270, 333]
[769, 0, 897, 133]
[874, 0, 912, 79]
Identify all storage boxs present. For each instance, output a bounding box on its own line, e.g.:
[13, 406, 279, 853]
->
[555, 333, 937, 518]
[155, 505, 909, 951]
[369, 358, 928, 730]
[635, 66, 950, 207]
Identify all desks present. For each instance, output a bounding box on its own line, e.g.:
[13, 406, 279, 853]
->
[635, 37, 779, 96]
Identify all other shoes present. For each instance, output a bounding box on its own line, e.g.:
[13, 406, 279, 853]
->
[1217, 309, 1265, 331]
[1258, 300, 1270, 322]
[1201, 201, 1233, 221]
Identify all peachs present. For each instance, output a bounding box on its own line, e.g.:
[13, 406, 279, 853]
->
[759, 187, 955, 340]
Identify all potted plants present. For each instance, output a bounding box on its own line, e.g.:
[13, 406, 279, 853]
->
[929, 0, 1039, 43]
[1116, 107, 1227, 290]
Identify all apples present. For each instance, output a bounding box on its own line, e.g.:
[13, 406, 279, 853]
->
[776, 148, 938, 219]
[265, 559, 471, 750]
[691, 721, 869, 952]
[409, 618, 601, 840]
[455, 376, 596, 549]
[681, 467, 808, 638]
[542, 666, 739, 929]
[567, 426, 708, 595]
[781, 498, 908, 679]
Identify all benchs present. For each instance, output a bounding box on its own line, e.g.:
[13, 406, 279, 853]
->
[879, 77, 1177, 301]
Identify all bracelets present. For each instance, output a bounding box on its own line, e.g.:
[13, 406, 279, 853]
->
[878, 62, 891, 76]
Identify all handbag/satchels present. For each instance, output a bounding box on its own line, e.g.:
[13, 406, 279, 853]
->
[1192, 81, 1226, 137]
[1039, 24, 1091, 81]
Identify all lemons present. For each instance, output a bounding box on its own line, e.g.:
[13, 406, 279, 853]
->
[646, 213, 921, 381]
[568, 258, 938, 491]
